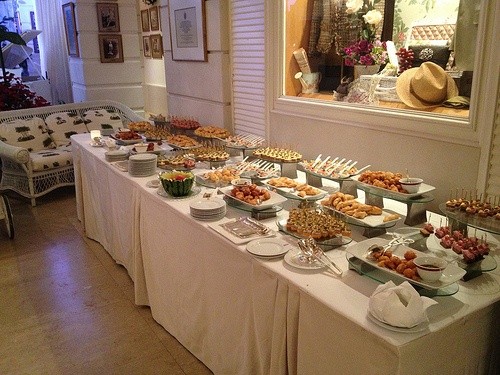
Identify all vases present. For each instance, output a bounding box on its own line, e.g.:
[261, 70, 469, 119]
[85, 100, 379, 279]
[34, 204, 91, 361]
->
[354, 65, 380, 79]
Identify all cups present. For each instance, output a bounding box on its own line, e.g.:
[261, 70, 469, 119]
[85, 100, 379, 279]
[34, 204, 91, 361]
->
[293, 47, 311, 74]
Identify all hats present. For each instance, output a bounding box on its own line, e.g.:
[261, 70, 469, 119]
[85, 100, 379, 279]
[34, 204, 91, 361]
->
[395, 61, 459, 109]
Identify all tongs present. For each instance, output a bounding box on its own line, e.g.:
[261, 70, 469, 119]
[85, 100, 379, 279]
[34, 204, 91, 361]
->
[297, 235, 343, 276]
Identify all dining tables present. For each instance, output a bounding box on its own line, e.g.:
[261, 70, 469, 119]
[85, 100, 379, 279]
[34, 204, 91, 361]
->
[70, 134, 500, 375]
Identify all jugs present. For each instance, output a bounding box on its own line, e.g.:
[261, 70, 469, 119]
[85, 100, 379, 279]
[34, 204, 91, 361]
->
[295, 71, 322, 94]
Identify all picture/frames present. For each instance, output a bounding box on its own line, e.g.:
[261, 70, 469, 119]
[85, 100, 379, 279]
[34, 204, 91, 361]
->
[140, 6, 163, 59]
[62, 2, 79, 56]
[97, 3, 121, 32]
[168, 0, 208, 63]
[98, 34, 124, 63]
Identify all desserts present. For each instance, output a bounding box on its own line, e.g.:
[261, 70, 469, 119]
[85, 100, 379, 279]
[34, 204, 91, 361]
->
[114, 114, 357, 204]
[287, 169, 500, 280]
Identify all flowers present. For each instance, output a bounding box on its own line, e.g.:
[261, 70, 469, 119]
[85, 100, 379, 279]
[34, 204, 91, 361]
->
[342, 0, 389, 67]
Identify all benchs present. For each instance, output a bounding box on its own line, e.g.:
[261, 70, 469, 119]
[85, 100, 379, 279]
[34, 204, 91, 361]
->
[0, 100, 156, 207]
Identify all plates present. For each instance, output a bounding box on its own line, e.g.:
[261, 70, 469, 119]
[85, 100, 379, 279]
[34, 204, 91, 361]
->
[166, 141, 203, 149]
[128, 153, 158, 177]
[367, 306, 429, 333]
[345, 237, 467, 289]
[350, 171, 435, 201]
[246, 238, 291, 259]
[317, 196, 401, 227]
[111, 160, 129, 172]
[219, 185, 291, 211]
[208, 217, 276, 245]
[89, 132, 173, 161]
[260, 178, 328, 201]
[190, 197, 227, 221]
[284, 247, 329, 269]
[147, 179, 201, 199]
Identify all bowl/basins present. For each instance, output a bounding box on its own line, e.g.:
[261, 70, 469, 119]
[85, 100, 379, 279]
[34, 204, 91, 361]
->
[230, 178, 251, 189]
[134, 143, 148, 153]
[118, 129, 132, 140]
[412, 256, 448, 283]
[399, 178, 423, 193]
